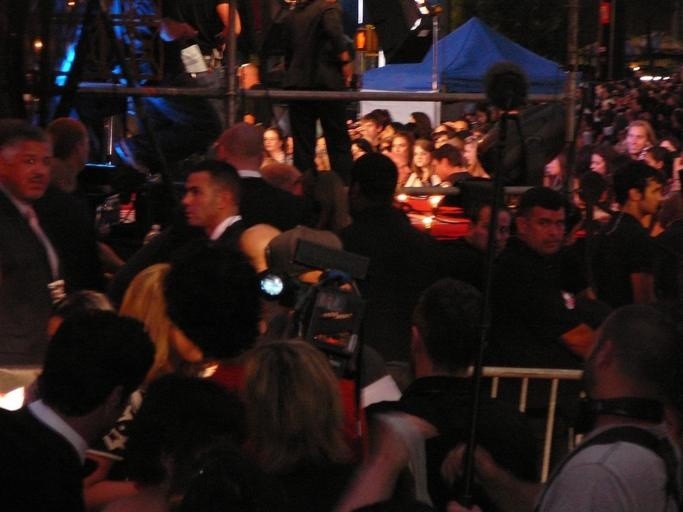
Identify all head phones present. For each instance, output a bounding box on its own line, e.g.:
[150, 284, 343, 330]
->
[567, 397, 662, 434]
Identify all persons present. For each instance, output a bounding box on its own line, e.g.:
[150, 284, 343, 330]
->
[1, 0, 681, 512]
